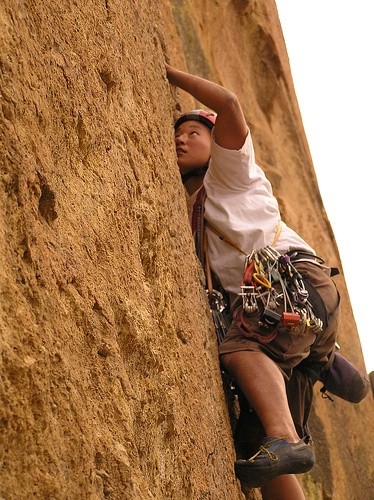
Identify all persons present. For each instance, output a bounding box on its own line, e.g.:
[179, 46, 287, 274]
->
[164, 62, 339, 500]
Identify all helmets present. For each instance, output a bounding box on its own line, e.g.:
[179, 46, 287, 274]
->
[174, 109, 217, 128]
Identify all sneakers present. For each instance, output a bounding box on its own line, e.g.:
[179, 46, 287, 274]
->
[234, 436, 316, 492]
